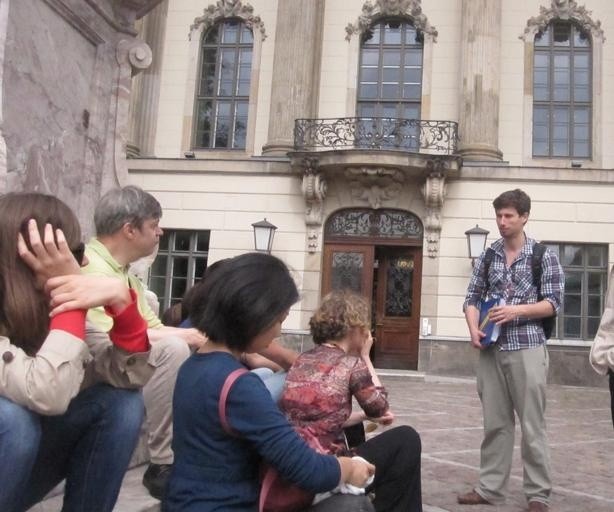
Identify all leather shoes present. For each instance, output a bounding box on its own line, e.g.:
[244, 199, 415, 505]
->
[143, 462, 173, 502]
[528, 501, 548, 511]
[457, 491, 485, 505]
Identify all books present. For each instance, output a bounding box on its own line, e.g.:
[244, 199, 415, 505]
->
[479, 297, 508, 345]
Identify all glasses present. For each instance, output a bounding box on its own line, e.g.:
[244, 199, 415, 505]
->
[72, 242, 85, 266]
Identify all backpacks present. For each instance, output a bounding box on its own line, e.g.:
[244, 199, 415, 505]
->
[483, 242, 554, 341]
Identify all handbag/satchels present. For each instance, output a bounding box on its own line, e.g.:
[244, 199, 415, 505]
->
[217, 366, 329, 511]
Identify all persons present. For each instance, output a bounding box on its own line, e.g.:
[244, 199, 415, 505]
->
[0, 192, 161, 512]
[141, 252, 428, 512]
[586, 264, 613, 429]
[78, 180, 210, 499]
[458, 188, 568, 512]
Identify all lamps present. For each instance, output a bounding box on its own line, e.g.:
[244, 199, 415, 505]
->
[464, 224, 489, 267]
[251, 215, 277, 255]
